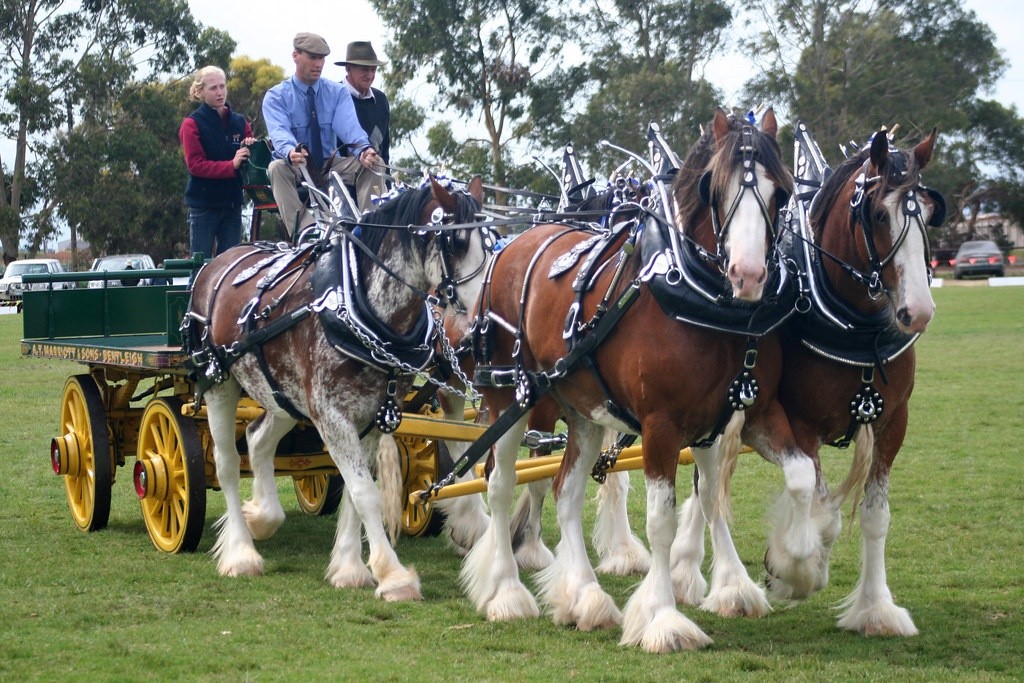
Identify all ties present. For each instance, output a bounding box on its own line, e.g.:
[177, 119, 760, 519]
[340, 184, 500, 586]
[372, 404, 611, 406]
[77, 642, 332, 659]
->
[307, 87, 324, 172]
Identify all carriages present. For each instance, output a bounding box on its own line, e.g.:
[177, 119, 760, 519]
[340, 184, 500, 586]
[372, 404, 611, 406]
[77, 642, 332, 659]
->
[17, 105, 948, 655]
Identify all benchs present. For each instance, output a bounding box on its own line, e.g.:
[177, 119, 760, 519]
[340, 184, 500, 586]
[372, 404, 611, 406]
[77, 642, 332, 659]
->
[241, 140, 358, 243]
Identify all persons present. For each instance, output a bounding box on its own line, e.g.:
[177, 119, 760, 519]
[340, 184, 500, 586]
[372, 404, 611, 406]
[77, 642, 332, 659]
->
[262, 32, 392, 243]
[179, 66, 258, 290]
[150, 263, 173, 285]
[120, 260, 140, 286]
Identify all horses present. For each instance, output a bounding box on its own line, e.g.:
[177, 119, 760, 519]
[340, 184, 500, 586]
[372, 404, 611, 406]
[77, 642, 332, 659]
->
[181, 173, 495, 603]
[456, 107, 938, 660]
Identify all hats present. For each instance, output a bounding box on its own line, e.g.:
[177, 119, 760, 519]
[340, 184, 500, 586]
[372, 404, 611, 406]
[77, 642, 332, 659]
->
[293, 32, 330, 56]
[334, 41, 388, 66]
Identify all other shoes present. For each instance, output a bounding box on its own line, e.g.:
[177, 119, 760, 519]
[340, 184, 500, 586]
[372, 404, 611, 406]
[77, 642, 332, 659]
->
[298, 226, 314, 246]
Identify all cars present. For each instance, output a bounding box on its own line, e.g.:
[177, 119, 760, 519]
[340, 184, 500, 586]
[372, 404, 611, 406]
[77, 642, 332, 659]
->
[0, 260, 76, 303]
[954, 239, 1005, 279]
[86, 254, 155, 289]
